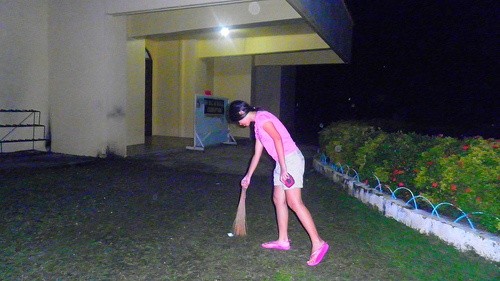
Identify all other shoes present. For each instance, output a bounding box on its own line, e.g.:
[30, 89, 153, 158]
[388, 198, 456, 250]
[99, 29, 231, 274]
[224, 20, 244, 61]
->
[260, 241, 290, 250]
[308, 242, 330, 267]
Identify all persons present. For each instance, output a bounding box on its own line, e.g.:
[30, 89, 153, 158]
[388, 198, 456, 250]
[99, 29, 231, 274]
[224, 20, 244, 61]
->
[228, 101, 329, 266]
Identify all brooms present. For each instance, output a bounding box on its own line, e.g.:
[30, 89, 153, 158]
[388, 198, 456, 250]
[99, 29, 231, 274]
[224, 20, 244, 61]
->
[229, 182, 247, 237]
